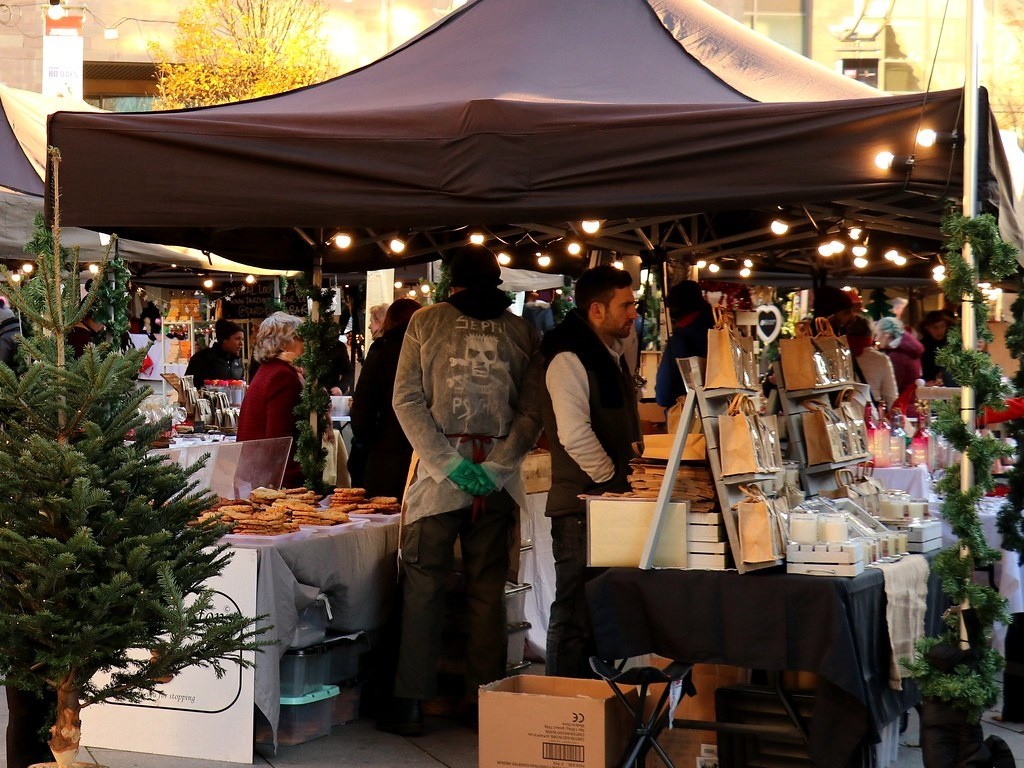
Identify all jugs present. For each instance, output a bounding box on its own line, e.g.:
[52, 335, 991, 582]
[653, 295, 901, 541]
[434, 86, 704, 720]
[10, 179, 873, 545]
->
[329, 396, 352, 416]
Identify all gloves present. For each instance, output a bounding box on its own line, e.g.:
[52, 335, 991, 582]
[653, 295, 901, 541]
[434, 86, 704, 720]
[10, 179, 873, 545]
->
[444, 452, 496, 496]
[600, 473, 630, 493]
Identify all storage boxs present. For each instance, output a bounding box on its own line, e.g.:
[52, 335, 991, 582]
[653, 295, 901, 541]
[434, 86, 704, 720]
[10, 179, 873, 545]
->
[686, 512, 730, 570]
[884, 524, 943, 552]
[255, 545, 821, 767]
[330, 396, 352, 416]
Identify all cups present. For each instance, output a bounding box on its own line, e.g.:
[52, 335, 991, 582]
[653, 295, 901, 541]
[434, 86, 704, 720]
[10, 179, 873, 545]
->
[878, 489, 930, 521]
[764, 459, 801, 492]
[788, 508, 849, 543]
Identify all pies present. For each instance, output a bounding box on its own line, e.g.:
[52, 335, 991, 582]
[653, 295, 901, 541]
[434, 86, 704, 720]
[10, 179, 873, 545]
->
[189, 486, 402, 538]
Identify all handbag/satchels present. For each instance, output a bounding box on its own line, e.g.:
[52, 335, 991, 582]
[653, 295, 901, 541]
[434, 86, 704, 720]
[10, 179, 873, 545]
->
[704, 314, 884, 561]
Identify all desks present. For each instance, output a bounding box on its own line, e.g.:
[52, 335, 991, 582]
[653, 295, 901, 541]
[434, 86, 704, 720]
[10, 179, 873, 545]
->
[330, 416, 351, 437]
[847, 464, 932, 499]
[82, 512, 403, 762]
[917, 385, 962, 400]
[128, 435, 238, 498]
[929, 495, 1024, 613]
[546, 545, 982, 767]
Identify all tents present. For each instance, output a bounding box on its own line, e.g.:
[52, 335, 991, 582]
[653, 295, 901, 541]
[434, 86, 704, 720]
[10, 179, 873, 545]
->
[43, 1, 1023, 652]
[0, 92, 299, 309]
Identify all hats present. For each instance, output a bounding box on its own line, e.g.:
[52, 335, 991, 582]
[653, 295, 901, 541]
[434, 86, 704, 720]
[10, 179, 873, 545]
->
[0, 308, 15, 323]
[215, 318, 245, 342]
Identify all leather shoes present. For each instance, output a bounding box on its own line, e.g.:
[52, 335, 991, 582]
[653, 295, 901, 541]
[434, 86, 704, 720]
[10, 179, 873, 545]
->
[377, 698, 425, 735]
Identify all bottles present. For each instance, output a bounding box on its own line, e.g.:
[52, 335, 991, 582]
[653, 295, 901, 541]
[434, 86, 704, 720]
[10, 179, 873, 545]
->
[862, 399, 936, 473]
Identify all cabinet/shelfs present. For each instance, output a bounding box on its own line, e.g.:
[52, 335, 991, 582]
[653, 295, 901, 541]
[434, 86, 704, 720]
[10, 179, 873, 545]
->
[773, 361, 872, 499]
[673, 356, 797, 575]
[161, 316, 250, 409]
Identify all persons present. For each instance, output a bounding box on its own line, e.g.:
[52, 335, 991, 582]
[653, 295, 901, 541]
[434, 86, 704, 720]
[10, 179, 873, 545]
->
[51, 246, 951, 736]
